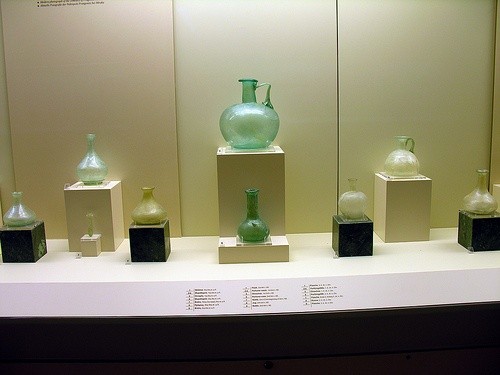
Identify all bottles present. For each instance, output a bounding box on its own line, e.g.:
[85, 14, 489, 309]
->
[129, 186, 168, 225]
[463, 169, 499, 215]
[74, 133, 108, 186]
[237, 189, 271, 242]
[3, 191, 36, 227]
[338, 178, 369, 221]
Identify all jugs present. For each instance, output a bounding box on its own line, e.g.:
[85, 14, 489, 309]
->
[382, 134, 421, 178]
[217, 78, 280, 150]
